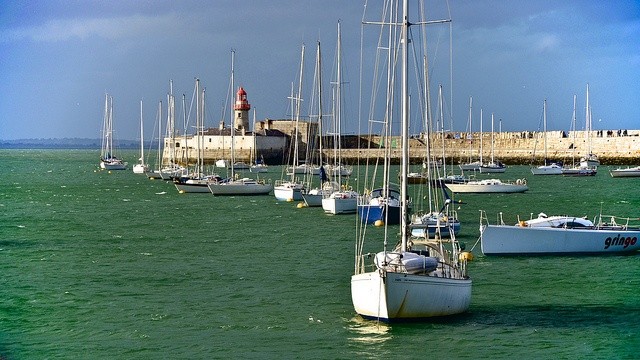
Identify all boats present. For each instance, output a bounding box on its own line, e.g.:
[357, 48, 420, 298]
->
[445, 178, 529, 193]
[609, 166, 640, 178]
[479, 210, 640, 254]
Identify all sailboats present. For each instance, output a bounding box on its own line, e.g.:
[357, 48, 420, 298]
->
[398, 95, 429, 184]
[530, 100, 562, 175]
[423, 122, 442, 168]
[159, 93, 188, 179]
[104, 97, 127, 170]
[274, 44, 310, 202]
[427, 84, 473, 188]
[251, 132, 268, 173]
[170, 94, 189, 178]
[459, 109, 487, 171]
[405, 39, 460, 238]
[229, 138, 250, 169]
[100, 94, 128, 168]
[580, 83, 600, 168]
[302, 40, 338, 207]
[321, 22, 362, 214]
[323, 102, 353, 176]
[175, 79, 231, 193]
[216, 130, 228, 167]
[358, 48, 407, 225]
[479, 113, 506, 173]
[144, 101, 162, 178]
[561, 95, 597, 176]
[207, 49, 273, 195]
[351, 0, 471, 319]
[288, 82, 320, 174]
[171, 90, 222, 184]
[322, 86, 339, 195]
[133, 100, 149, 174]
